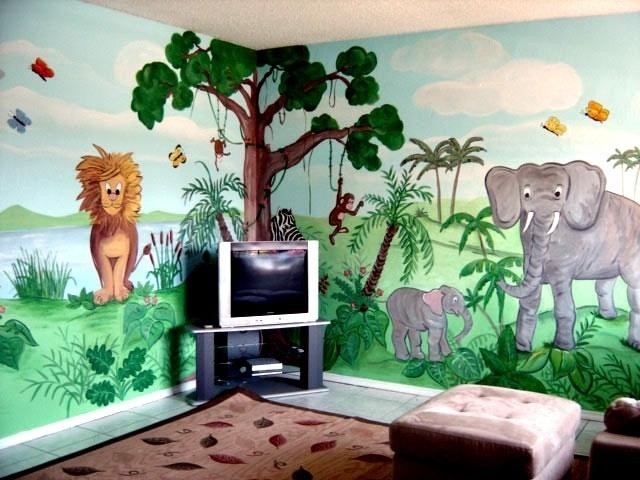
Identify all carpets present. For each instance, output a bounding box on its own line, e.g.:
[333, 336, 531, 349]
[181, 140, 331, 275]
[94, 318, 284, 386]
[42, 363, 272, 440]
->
[0, 387, 589, 480]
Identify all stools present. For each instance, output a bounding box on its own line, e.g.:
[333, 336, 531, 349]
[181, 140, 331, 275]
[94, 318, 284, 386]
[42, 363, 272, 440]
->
[389, 384, 582, 480]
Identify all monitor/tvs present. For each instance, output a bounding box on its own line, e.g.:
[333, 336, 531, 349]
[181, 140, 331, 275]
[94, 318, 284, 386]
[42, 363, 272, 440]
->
[219, 240, 318, 328]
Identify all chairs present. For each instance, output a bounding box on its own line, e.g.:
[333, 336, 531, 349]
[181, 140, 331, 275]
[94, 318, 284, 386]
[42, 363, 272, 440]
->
[587, 396, 640, 480]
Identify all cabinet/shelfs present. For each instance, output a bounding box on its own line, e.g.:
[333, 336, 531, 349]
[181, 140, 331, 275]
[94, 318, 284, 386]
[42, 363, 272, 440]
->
[184, 320, 332, 405]
